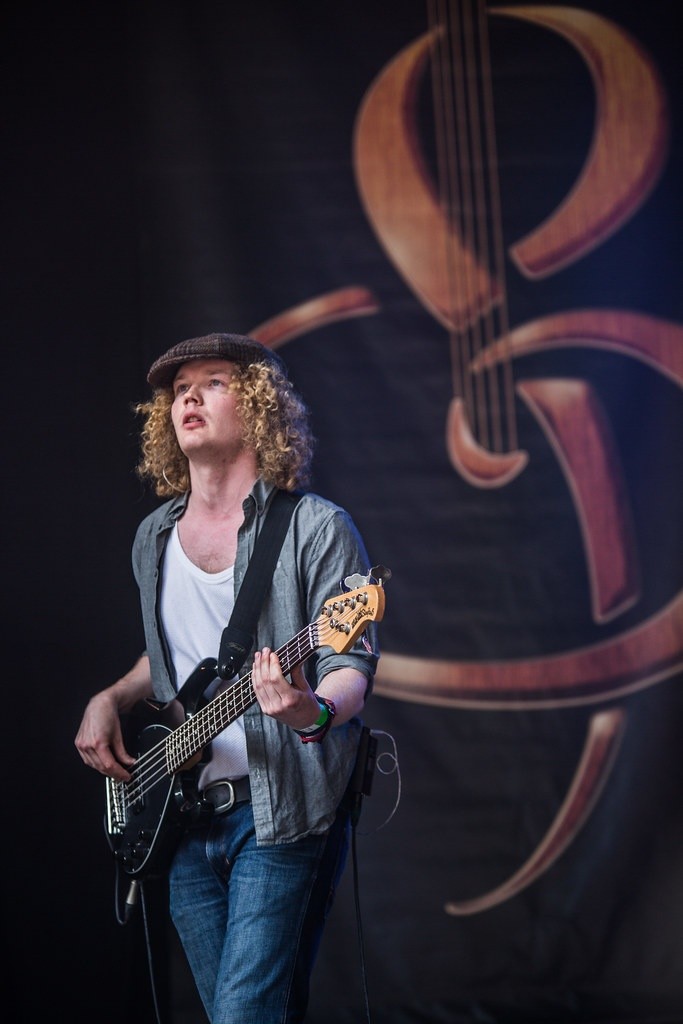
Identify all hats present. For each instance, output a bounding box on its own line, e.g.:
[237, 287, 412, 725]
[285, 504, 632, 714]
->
[147, 334, 287, 386]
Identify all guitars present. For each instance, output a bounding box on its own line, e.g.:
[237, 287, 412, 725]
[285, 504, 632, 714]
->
[102, 561, 395, 878]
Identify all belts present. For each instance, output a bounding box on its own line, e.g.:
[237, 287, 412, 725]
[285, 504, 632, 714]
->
[188, 775, 251, 816]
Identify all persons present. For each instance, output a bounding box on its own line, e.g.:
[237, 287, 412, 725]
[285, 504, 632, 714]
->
[74, 333, 381, 1024]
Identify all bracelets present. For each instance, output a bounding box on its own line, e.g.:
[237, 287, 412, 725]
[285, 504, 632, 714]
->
[294, 693, 337, 745]
